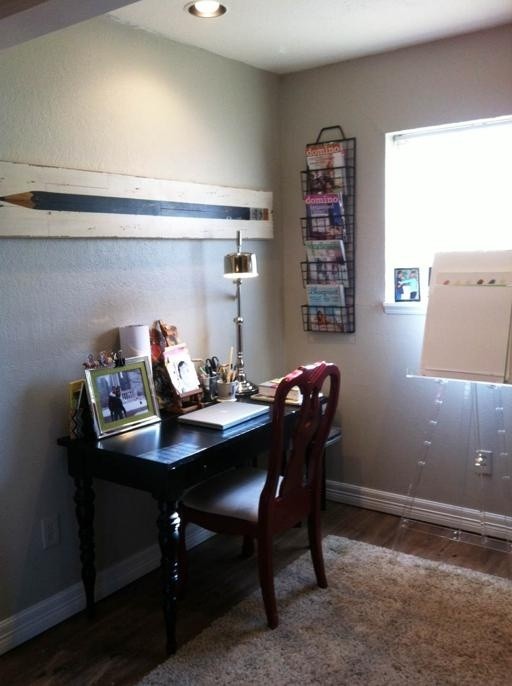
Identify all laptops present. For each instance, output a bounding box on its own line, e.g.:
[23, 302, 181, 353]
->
[177, 400, 270, 431]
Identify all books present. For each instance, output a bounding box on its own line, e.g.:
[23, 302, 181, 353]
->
[250, 378, 324, 406]
[304, 142, 350, 332]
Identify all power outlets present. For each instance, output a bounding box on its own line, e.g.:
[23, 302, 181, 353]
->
[475, 447, 493, 476]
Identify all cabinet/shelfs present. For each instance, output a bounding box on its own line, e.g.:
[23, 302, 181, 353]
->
[295, 125, 356, 335]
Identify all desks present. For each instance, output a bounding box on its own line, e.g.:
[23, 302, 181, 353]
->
[54, 389, 330, 656]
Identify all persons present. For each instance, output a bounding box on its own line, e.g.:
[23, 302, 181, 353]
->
[400, 270, 418, 298]
[396, 271, 405, 299]
[107, 386, 127, 422]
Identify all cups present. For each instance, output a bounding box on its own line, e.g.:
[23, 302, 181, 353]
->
[217, 380, 239, 399]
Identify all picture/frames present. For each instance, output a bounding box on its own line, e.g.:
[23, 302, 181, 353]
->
[394, 268, 419, 301]
[85, 354, 163, 443]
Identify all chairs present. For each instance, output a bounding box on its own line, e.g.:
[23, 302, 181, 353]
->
[177, 362, 343, 631]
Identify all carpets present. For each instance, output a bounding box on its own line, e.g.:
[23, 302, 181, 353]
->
[133, 534, 511, 685]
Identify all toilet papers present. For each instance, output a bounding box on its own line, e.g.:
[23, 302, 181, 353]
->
[120, 324, 153, 374]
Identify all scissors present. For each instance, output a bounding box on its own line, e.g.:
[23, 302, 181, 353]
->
[206, 356, 220, 376]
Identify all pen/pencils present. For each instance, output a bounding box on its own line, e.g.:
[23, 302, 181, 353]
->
[199, 346, 237, 384]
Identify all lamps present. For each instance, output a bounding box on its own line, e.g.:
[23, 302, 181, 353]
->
[221, 255, 260, 398]
